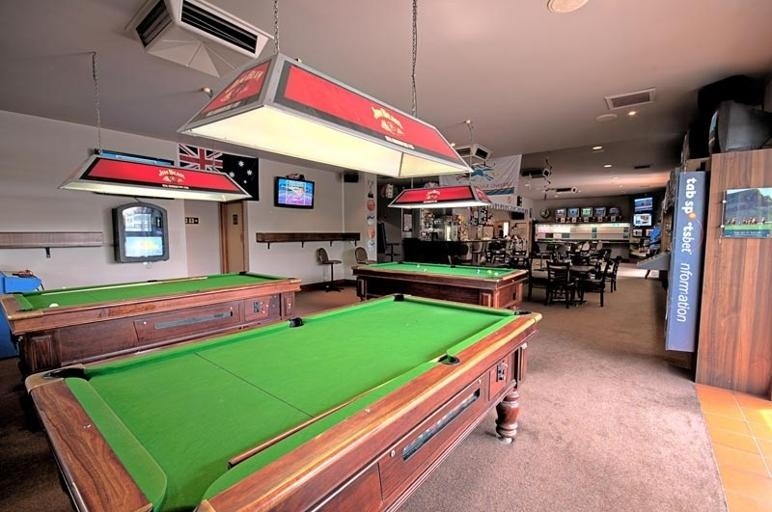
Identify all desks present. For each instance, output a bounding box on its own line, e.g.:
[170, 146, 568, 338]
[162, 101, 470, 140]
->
[0, 271, 301, 431]
[25, 294, 544, 512]
[352, 260, 529, 311]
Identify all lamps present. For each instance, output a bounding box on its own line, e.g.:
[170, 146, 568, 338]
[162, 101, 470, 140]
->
[176, 0, 475, 180]
[388, 119, 493, 210]
[57, 52, 252, 202]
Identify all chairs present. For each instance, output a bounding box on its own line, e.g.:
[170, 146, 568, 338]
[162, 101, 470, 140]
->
[529, 241, 621, 308]
[355, 247, 377, 264]
[318, 248, 345, 292]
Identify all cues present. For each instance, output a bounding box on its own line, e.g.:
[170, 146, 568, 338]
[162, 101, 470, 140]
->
[228, 353, 449, 467]
[44, 276, 207, 296]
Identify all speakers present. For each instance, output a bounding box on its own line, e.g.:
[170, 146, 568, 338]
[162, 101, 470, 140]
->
[344, 174, 359, 183]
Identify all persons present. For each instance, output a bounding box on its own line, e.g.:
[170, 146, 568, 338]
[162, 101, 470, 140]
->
[725, 216, 766, 225]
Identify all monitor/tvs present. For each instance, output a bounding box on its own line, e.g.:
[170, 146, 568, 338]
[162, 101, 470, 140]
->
[95, 148, 174, 200]
[274, 176, 314, 208]
[632, 213, 653, 227]
[634, 197, 654, 213]
